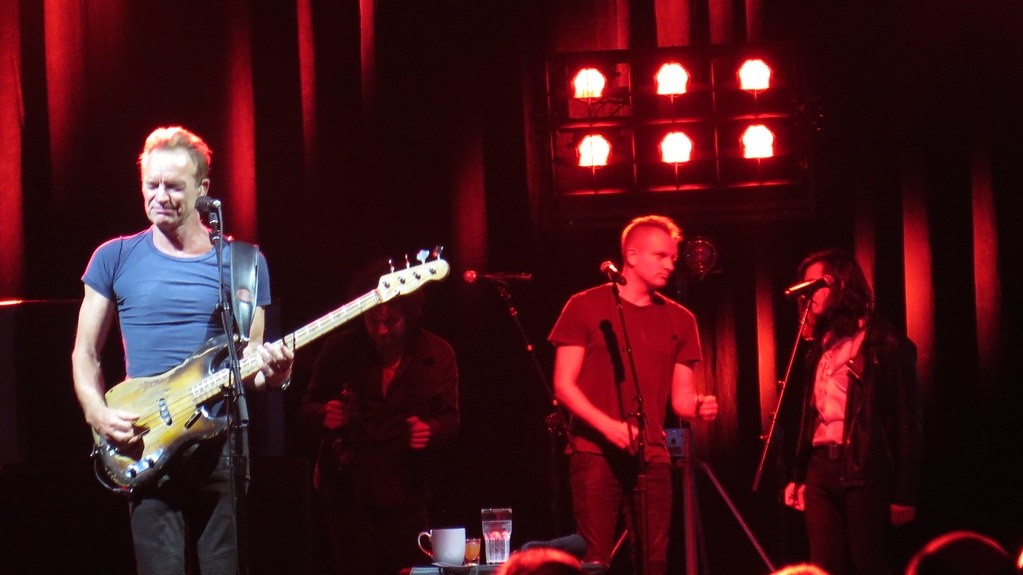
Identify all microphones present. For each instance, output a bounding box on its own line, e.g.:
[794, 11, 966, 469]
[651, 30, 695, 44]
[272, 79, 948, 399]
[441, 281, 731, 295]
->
[600, 261, 627, 286]
[196, 196, 222, 212]
[784, 275, 835, 300]
[463, 270, 532, 284]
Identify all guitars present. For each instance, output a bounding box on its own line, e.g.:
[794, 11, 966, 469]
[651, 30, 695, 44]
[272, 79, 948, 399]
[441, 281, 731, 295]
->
[89, 243, 452, 493]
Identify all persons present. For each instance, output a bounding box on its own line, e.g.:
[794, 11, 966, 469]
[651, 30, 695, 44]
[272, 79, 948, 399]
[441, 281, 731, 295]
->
[784, 250, 919, 574]
[71, 123, 294, 575]
[491, 530, 1023, 575]
[550, 212, 719, 574]
[303, 263, 457, 575]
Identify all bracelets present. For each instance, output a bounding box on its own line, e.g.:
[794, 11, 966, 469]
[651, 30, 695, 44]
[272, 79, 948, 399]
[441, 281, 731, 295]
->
[695, 403, 701, 419]
[265, 379, 291, 393]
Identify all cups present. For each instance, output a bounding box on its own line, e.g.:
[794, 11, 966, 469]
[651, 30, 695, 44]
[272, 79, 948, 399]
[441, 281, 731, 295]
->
[418, 528, 466, 567]
[463, 538, 481, 567]
[481, 508, 512, 565]
